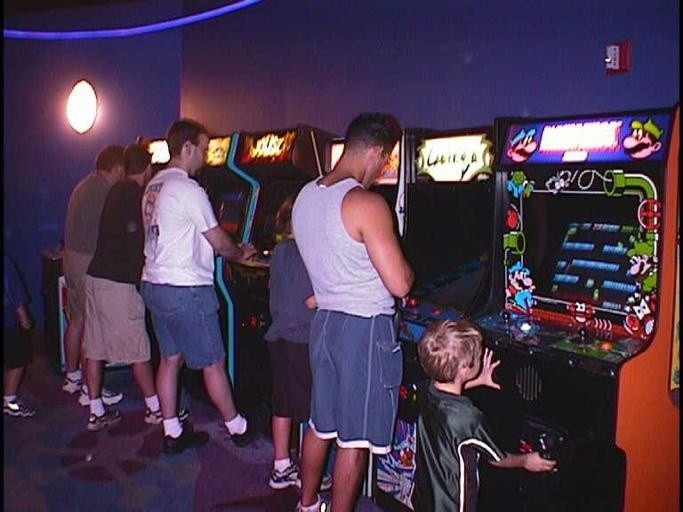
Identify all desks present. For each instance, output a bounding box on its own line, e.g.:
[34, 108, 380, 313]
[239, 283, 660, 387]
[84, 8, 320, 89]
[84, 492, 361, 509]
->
[37, 248, 132, 379]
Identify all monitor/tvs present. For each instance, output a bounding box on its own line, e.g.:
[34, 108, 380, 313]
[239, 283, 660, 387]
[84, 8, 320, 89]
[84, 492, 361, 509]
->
[503, 173, 657, 330]
[405, 182, 495, 312]
[252, 177, 301, 257]
[213, 183, 248, 245]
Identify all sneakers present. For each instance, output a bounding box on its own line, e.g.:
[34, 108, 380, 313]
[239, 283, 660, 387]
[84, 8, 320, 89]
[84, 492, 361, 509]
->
[61, 369, 84, 394]
[145, 407, 190, 424]
[87, 409, 121, 432]
[230, 403, 274, 450]
[293, 495, 330, 512]
[163, 427, 210, 456]
[77, 383, 125, 408]
[268, 464, 332, 491]
[2, 397, 38, 417]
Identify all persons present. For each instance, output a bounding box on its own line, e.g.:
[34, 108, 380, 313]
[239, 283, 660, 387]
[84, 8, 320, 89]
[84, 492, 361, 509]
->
[291, 112, 415, 512]
[412, 318, 556, 512]
[61, 145, 125, 408]
[264, 199, 332, 491]
[82, 144, 190, 431]
[140, 119, 271, 453]
[3, 256, 35, 418]
